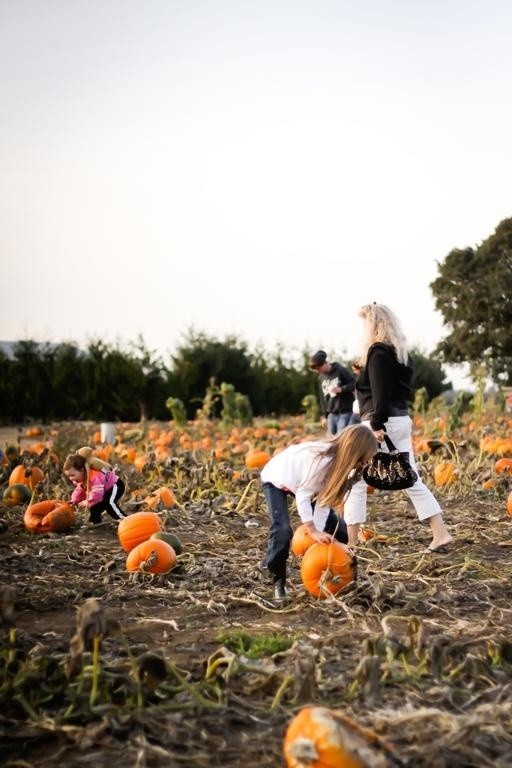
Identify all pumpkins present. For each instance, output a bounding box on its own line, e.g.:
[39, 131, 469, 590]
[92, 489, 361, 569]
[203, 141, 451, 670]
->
[0, 413, 512, 515]
[118, 512, 164, 554]
[24, 500, 75, 533]
[125, 539, 175, 573]
[293, 525, 316, 555]
[301, 541, 355, 597]
[283, 706, 370, 768]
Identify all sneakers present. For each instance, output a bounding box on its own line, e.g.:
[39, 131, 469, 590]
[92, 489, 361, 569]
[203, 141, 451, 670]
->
[273, 579, 285, 598]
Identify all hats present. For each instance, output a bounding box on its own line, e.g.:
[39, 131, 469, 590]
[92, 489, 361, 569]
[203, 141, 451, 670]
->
[310, 350, 326, 367]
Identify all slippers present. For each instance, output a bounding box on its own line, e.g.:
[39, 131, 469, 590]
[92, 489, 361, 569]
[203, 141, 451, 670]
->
[420, 546, 450, 555]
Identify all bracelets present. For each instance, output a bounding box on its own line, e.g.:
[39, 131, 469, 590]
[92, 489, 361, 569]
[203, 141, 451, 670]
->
[307, 523, 314, 528]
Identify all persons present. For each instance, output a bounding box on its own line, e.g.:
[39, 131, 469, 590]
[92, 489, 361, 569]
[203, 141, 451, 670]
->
[310, 349, 356, 436]
[343, 301, 453, 555]
[259, 423, 380, 601]
[62, 453, 127, 524]
[347, 364, 361, 426]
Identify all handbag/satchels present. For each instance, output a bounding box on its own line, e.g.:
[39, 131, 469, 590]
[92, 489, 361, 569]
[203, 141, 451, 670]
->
[363, 451, 417, 490]
